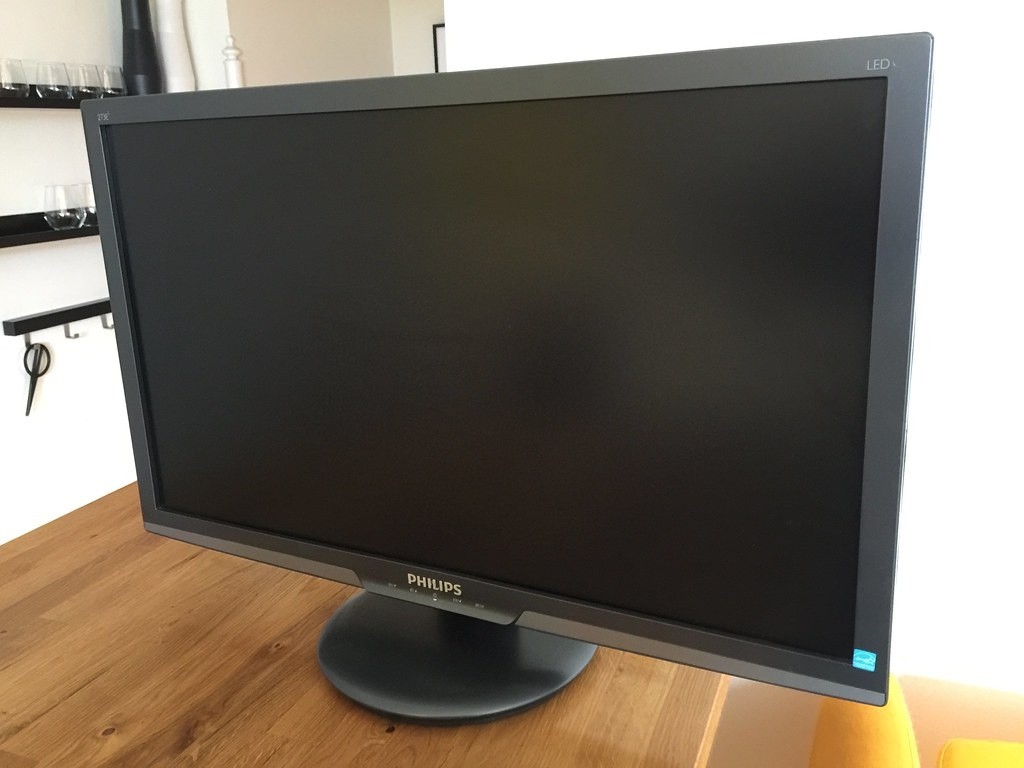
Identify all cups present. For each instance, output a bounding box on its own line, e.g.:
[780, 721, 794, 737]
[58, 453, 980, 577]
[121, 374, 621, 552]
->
[35, 59, 71, 99]
[71, 63, 105, 99]
[72, 186, 100, 228]
[0, 59, 31, 99]
[100, 66, 129, 97]
[42, 185, 87, 230]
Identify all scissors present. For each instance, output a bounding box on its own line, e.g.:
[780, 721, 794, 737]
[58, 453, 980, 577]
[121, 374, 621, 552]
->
[23, 343, 51, 417]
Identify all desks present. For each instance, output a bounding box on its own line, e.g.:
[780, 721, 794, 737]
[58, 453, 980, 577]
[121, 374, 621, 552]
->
[1, 479, 735, 768]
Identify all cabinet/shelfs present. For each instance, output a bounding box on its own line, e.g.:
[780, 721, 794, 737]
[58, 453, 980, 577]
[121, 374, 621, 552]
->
[0, 82, 128, 337]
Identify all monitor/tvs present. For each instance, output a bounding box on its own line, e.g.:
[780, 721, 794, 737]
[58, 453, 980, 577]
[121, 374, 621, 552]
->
[80, 30, 936, 726]
